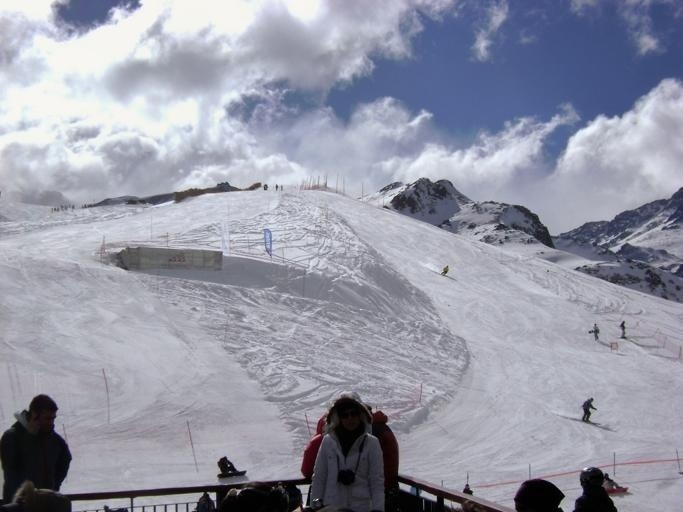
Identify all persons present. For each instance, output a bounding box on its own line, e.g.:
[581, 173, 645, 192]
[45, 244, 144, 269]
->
[514, 478, 566, 512]
[366, 404, 400, 511]
[302, 414, 326, 506]
[573, 466, 618, 512]
[582, 398, 597, 422]
[604, 473, 620, 490]
[463, 484, 472, 494]
[310, 397, 387, 511]
[441, 265, 449, 276]
[620, 321, 626, 338]
[1, 394, 71, 506]
[592, 323, 600, 340]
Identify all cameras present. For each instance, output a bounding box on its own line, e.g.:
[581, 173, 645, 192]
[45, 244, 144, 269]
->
[338, 469, 355, 485]
